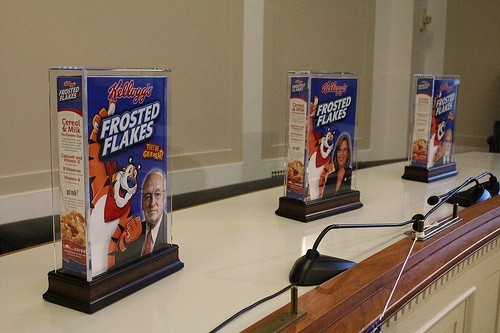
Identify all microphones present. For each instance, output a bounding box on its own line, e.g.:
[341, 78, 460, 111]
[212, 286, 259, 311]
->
[427, 174, 500, 208]
[287, 214, 427, 286]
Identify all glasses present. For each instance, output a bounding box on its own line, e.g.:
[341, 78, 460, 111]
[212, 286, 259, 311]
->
[143, 190, 166, 201]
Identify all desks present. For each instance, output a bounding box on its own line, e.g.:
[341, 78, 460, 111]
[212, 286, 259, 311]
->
[0, 150, 499, 333]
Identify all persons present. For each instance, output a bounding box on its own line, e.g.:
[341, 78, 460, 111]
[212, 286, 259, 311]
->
[439, 129, 453, 166]
[322, 133, 352, 200]
[115, 170, 166, 263]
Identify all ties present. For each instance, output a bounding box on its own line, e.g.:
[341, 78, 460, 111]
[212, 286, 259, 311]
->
[143, 232, 152, 255]
